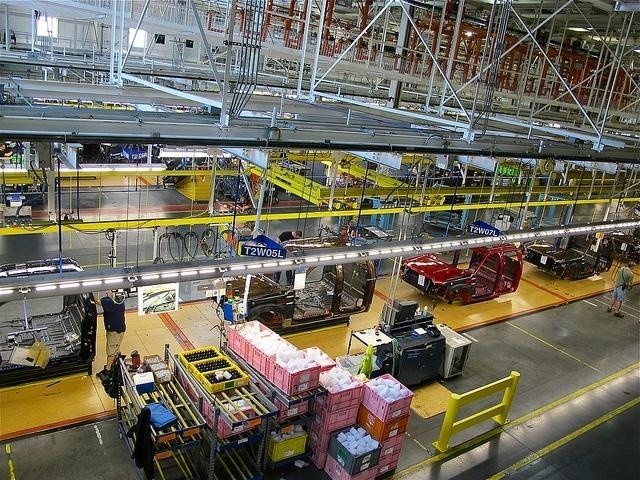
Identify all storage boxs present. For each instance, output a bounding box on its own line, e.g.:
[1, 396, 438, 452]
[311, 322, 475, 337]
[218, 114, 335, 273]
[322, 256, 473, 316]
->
[177, 318, 415, 480]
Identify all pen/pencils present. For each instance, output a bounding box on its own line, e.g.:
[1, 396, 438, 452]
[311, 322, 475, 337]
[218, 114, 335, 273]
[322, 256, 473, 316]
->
[396, 336, 404, 338]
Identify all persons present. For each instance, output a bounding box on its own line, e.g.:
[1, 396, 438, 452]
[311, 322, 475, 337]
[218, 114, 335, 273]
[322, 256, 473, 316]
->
[95, 289, 126, 377]
[273, 230, 302, 287]
[607, 261, 636, 318]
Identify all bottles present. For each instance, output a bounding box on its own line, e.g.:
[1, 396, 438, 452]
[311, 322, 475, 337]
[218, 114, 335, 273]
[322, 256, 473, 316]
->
[226, 283, 240, 303]
[423, 305, 427, 316]
[415, 305, 420, 314]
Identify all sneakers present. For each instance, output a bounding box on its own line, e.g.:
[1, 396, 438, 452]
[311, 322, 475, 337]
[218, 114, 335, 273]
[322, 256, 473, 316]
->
[96, 365, 112, 377]
[614, 312, 624, 317]
[607, 307, 611, 312]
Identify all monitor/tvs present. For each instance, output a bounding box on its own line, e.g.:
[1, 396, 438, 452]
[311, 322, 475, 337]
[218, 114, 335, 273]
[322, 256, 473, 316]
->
[393, 298, 419, 322]
